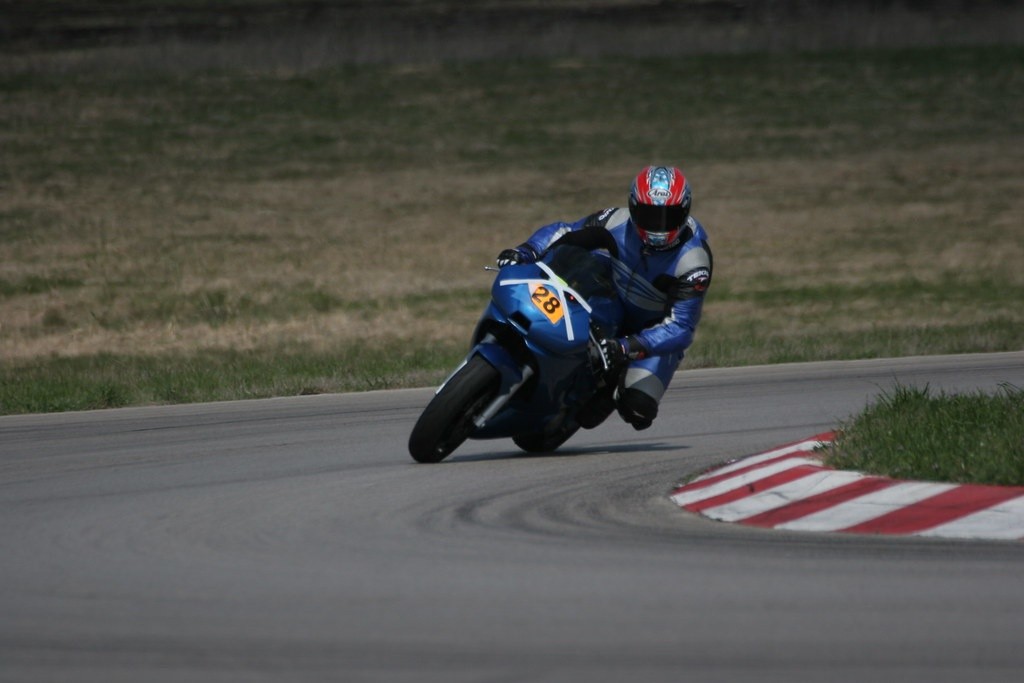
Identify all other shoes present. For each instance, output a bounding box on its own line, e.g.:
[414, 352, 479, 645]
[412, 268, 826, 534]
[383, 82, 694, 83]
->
[576, 380, 616, 429]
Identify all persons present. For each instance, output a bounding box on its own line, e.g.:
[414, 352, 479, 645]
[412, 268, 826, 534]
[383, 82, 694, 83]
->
[497, 163, 712, 431]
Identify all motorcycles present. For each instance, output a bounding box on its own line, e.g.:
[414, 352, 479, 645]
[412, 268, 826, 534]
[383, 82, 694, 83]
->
[408, 244, 616, 464]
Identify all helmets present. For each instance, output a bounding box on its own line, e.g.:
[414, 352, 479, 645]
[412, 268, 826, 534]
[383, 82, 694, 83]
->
[628, 166, 692, 246]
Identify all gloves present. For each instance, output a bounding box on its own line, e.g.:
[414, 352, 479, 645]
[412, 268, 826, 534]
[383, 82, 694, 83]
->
[600, 339, 621, 364]
[497, 249, 519, 269]
